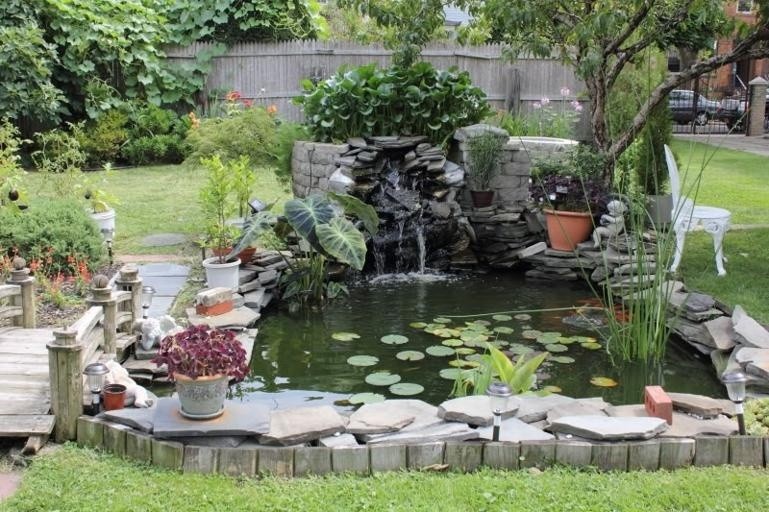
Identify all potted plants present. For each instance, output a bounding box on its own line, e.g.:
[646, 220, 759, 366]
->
[85, 200, 116, 249]
[530, 171, 609, 251]
[469, 133, 502, 208]
[151, 325, 251, 419]
[197, 152, 257, 293]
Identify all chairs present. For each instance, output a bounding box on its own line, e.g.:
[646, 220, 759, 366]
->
[662, 144, 733, 279]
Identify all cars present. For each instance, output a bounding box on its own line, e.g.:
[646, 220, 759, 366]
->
[719, 89, 768, 133]
[665, 90, 722, 126]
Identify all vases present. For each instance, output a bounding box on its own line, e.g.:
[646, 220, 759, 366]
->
[104, 383, 127, 410]
